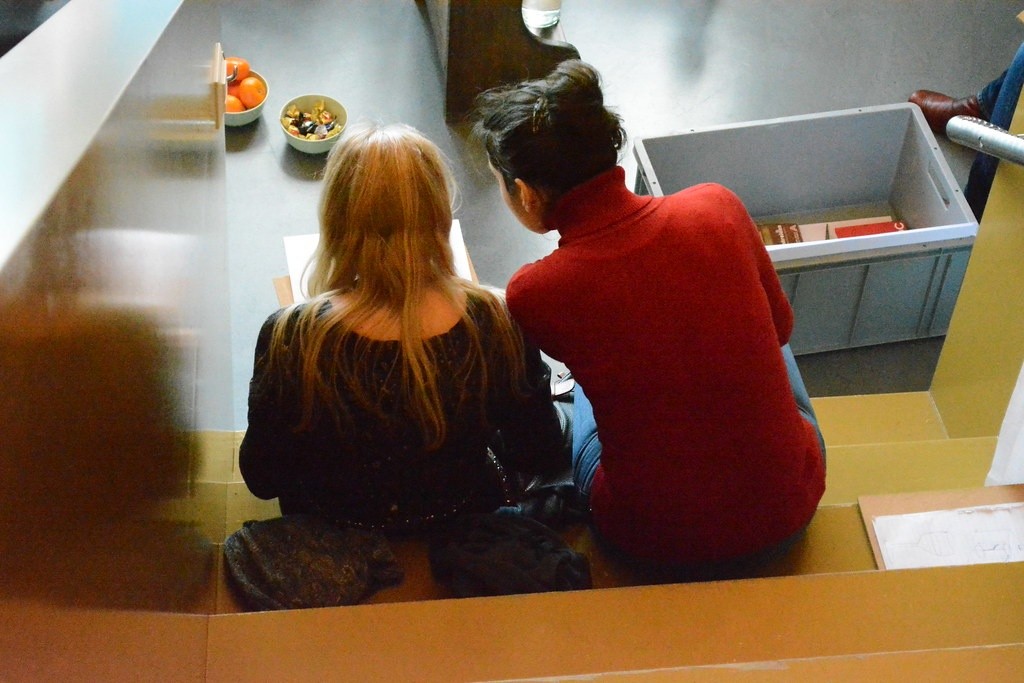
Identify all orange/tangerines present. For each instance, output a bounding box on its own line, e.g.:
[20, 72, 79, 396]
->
[225, 56, 266, 113]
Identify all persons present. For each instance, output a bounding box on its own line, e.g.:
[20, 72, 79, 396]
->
[238, 122, 571, 544]
[470, 58, 828, 579]
[908, 42, 1024, 225]
[0, 146, 217, 607]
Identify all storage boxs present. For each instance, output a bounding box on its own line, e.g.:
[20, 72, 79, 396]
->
[633, 103, 981, 355]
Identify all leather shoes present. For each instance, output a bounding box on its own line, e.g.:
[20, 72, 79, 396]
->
[909, 90, 983, 134]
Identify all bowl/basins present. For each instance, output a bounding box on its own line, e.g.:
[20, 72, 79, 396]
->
[225, 68, 269, 128]
[279, 94, 349, 153]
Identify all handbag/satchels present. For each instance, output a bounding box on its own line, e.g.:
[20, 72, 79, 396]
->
[510, 370, 590, 526]
